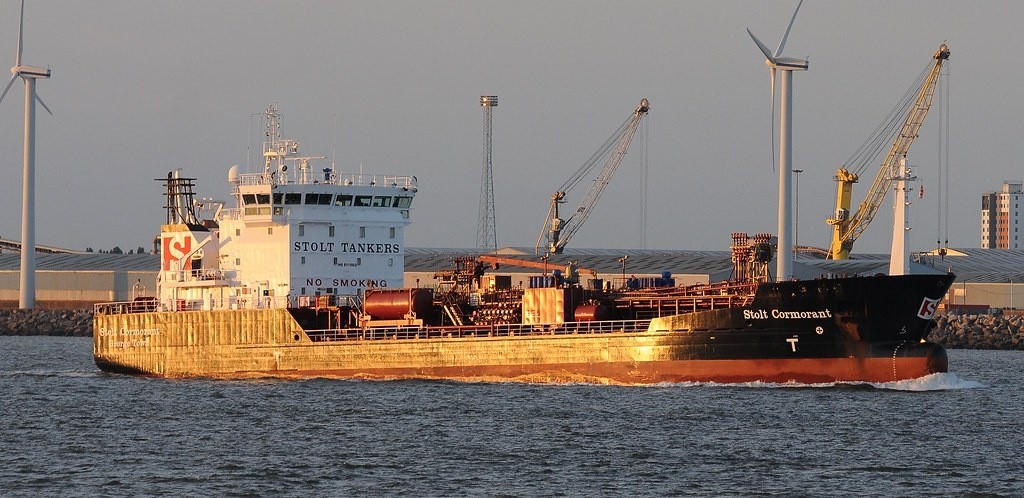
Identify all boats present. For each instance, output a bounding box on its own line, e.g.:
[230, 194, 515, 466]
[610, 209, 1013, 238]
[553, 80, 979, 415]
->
[88, 99, 960, 389]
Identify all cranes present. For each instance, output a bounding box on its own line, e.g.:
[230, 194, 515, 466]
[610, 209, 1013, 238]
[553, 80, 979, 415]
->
[826, 39, 957, 257]
[534, 97, 650, 257]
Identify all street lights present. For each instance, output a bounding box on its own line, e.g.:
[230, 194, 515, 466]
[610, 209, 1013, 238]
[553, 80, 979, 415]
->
[791, 168, 804, 260]
